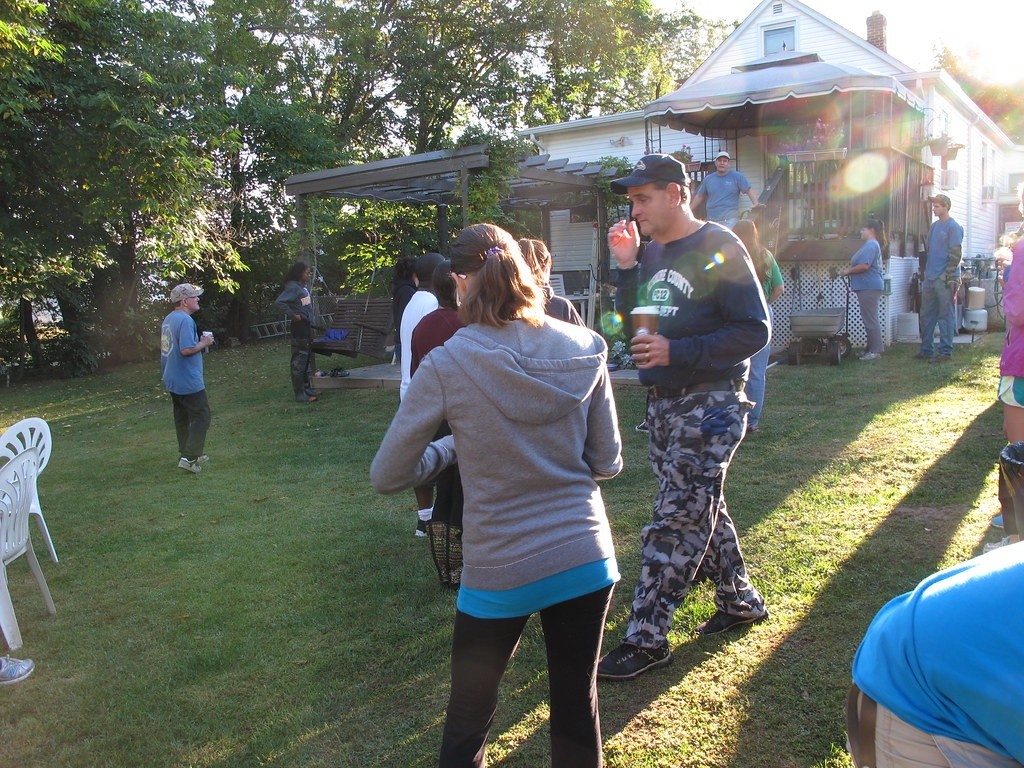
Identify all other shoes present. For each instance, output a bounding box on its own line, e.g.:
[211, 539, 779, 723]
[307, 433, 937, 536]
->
[0, 655, 35, 685]
[928, 354, 951, 363]
[177, 457, 201, 473]
[991, 514, 1004, 530]
[910, 352, 928, 359]
[855, 349, 868, 357]
[330, 368, 350, 378]
[636, 419, 648, 432]
[859, 351, 881, 361]
[197, 455, 209, 465]
[414, 518, 431, 538]
[747, 424, 760, 434]
[982, 535, 1018, 553]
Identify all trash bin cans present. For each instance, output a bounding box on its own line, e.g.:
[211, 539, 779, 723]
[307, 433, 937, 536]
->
[1000, 442, 1024, 541]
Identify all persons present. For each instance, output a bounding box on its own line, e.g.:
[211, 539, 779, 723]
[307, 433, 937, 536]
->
[733, 219, 784, 429]
[690, 151, 767, 232]
[365, 223, 626, 768]
[387, 253, 471, 538]
[838, 216, 885, 360]
[598, 151, 774, 681]
[846, 182, 1024, 768]
[158, 282, 214, 472]
[910, 194, 965, 362]
[272, 260, 322, 400]
[633, 395, 649, 433]
[516, 237, 587, 328]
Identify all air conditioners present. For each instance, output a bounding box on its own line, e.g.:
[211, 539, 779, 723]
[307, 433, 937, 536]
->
[940, 170, 960, 190]
[982, 186, 1000, 203]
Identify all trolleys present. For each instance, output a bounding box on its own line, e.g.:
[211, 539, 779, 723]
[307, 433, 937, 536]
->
[788, 275, 851, 367]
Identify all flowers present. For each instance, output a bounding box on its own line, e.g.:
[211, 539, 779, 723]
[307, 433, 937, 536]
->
[778, 117, 847, 152]
[667, 144, 693, 164]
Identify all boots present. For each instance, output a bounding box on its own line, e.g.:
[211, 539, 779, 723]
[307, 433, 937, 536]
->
[293, 376, 317, 403]
[446, 523, 467, 589]
[429, 522, 447, 584]
[301, 382, 323, 396]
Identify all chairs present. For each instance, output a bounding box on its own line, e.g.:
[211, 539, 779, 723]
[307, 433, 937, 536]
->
[548, 274, 568, 297]
[0, 447, 57, 651]
[0, 418, 59, 562]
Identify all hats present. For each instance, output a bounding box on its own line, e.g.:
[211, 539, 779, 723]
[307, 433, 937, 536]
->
[714, 151, 730, 160]
[610, 154, 691, 195]
[171, 283, 205, 304]
[928, 195, 951, 209]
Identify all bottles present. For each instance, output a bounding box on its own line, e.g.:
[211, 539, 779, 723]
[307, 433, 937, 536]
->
[583, 289, 589, 295]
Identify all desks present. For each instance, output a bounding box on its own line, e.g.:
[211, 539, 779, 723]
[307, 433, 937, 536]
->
[563, 293, 600, 324]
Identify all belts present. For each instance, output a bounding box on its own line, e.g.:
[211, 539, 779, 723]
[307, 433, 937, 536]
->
[648, 379, 744, 398]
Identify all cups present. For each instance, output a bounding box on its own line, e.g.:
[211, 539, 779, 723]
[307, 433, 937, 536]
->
[631, 306, 659, 338]
[202, 331, 212, 337]
[574, 291, 580, 296]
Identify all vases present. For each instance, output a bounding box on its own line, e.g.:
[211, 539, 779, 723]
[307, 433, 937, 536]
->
[684, 161, 701, 173]
[785, 147, 847, 163]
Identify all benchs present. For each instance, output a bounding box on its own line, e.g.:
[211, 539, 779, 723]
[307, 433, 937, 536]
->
[309, 298, 393, 362]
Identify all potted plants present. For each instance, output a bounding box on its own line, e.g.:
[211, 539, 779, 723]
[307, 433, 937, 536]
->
[929, 135, 950, 155]
[946, 144, 966, 160]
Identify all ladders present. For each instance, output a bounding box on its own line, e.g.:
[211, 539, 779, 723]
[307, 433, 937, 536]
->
[250, 313, 335, 340]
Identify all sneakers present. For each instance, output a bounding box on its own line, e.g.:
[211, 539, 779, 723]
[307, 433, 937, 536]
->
[691, 610, 764, 637]
[598, 643, 673, 679]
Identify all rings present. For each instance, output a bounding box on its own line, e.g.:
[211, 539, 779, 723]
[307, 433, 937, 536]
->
[644, 344, 649, 352]
[645, 353, 650, 361]
